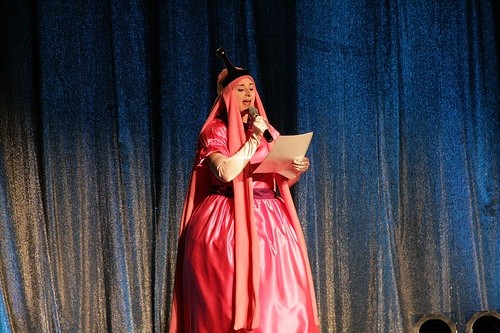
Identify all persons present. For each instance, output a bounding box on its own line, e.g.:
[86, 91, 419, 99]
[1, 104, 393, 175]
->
[168, 66, 321, 333]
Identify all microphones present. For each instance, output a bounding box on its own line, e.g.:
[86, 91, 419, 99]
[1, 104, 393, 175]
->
[249, 106, 273, 143]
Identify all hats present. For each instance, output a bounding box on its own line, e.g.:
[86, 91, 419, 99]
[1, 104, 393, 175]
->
[215, 66, 252, 94]
